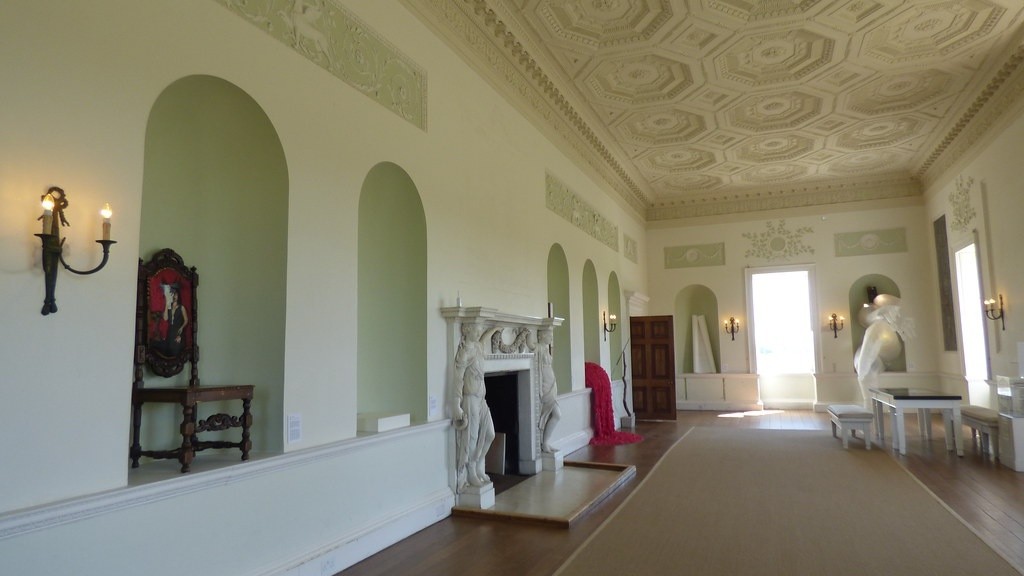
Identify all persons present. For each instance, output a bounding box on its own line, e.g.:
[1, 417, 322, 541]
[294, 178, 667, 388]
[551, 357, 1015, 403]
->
[450, 321, 495, 488]
[163, 284, 189, 342]
[537, 330, 563, 453]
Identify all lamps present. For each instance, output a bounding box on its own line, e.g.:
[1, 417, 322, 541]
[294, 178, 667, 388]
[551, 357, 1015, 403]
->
[724, 316, 740, 341]
[829, 313, 844, 338]
[33, 186, 118, 316]
[983, 294, 1006, 332]
[602, 311, 617, 342]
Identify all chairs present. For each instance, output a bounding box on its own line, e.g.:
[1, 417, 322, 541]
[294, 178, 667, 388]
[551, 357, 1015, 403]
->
[129, 249, 255, 473]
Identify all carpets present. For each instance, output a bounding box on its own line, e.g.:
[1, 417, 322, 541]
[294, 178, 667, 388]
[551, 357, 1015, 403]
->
[549, 425, 1024, 576]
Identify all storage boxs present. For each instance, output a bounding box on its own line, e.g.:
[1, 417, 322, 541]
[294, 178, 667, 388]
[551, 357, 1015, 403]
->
[996, 375, 1024, 418]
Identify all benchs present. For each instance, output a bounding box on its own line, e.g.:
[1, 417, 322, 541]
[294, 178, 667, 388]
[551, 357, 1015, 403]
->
[826, 403, 875, 450]
[949, 405, 1001, 459]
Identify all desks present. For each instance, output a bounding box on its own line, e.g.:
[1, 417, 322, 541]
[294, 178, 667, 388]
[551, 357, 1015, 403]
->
[868, 386, 966, 457]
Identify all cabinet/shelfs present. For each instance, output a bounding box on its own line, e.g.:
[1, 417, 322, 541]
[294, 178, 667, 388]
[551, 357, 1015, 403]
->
[996, 410, 1024, 473]
